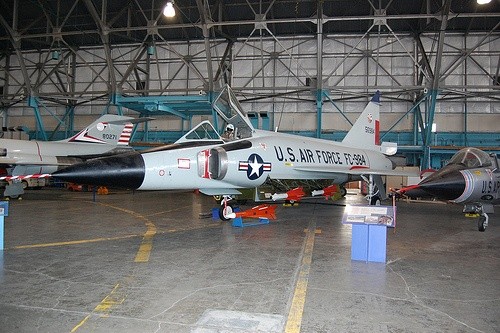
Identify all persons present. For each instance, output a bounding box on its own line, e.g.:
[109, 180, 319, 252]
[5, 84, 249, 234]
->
[219, 123, 235, 142]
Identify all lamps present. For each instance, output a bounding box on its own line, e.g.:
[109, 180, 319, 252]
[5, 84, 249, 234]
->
[162, 1, 177, 17]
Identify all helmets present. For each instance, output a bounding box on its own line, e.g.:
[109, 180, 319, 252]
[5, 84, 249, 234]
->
[225, 124, 234, 133]
[489, 152, 496, 158]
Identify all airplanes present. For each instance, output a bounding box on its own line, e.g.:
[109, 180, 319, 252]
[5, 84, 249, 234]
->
[0, 113, 137, 191]
[418, 147, 500, 232]
[46, 84, 398, 222]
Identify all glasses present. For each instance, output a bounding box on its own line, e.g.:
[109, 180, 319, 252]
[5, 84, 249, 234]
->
[227, 128, 233, 131]
